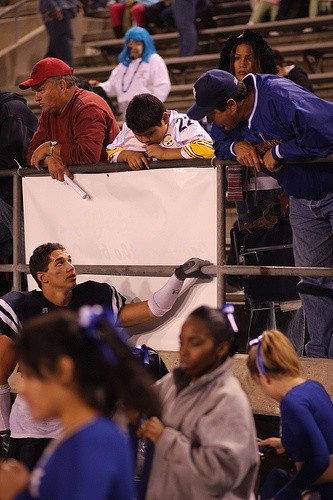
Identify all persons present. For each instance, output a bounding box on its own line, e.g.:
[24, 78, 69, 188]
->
[26, 0, 333, 184]
[186, 69, 333, 359]
[216, 29, 316, 354]
[0, 241, 332, 500]
[0, 88, 39, 295]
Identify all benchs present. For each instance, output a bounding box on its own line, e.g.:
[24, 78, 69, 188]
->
[25, 0, 333, 311]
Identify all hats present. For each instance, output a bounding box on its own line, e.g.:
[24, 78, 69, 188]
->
[188, 69, 238, 126]
[18, 57, 73, 93]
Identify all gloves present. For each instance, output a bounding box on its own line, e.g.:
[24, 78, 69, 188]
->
[175, 257, 212, 280]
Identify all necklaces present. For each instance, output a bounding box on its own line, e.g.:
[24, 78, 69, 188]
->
[121, 57, 145, 93]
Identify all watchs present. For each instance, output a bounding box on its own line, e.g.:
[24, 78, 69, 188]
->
[49, 140, 57, 157]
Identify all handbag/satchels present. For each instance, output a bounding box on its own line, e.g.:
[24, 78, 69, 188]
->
[226, 215, 299, 303]
